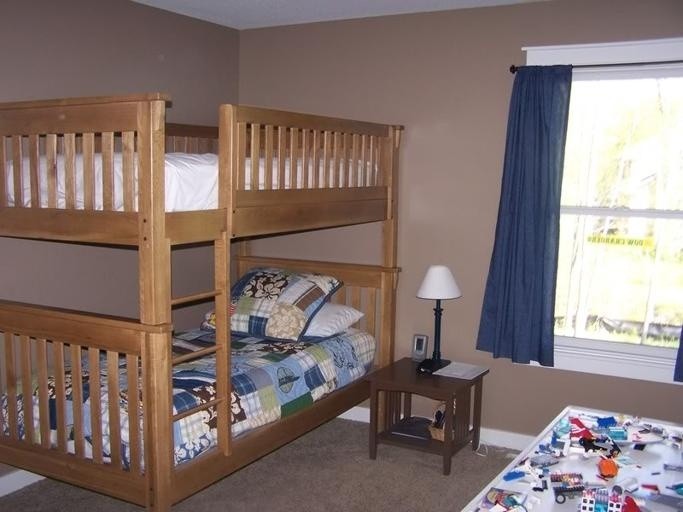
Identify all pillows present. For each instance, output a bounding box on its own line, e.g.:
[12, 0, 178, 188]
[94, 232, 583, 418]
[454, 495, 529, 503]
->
[200, 266, 344, 346]
[303, 302, 365, 338]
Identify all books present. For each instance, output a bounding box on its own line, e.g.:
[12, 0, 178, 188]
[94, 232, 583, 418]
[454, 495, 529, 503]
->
[432, 362, 488, 380]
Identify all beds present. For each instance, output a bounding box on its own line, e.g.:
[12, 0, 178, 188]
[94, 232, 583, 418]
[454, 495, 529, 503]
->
[1, 91, 405, 512]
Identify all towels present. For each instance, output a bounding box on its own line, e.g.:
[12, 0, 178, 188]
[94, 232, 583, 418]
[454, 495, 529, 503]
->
[432, 361, 489, 380]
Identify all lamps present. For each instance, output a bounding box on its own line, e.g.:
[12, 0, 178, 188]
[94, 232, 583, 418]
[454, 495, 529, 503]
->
[415, 265, 463, 374]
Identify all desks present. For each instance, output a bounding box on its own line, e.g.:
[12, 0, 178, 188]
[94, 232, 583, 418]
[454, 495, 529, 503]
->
[364, 357, 489, 476]
[460, 405, 683, 512]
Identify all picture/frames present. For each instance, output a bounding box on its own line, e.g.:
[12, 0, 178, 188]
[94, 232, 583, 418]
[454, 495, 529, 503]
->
[411, 334, 428, 362]
